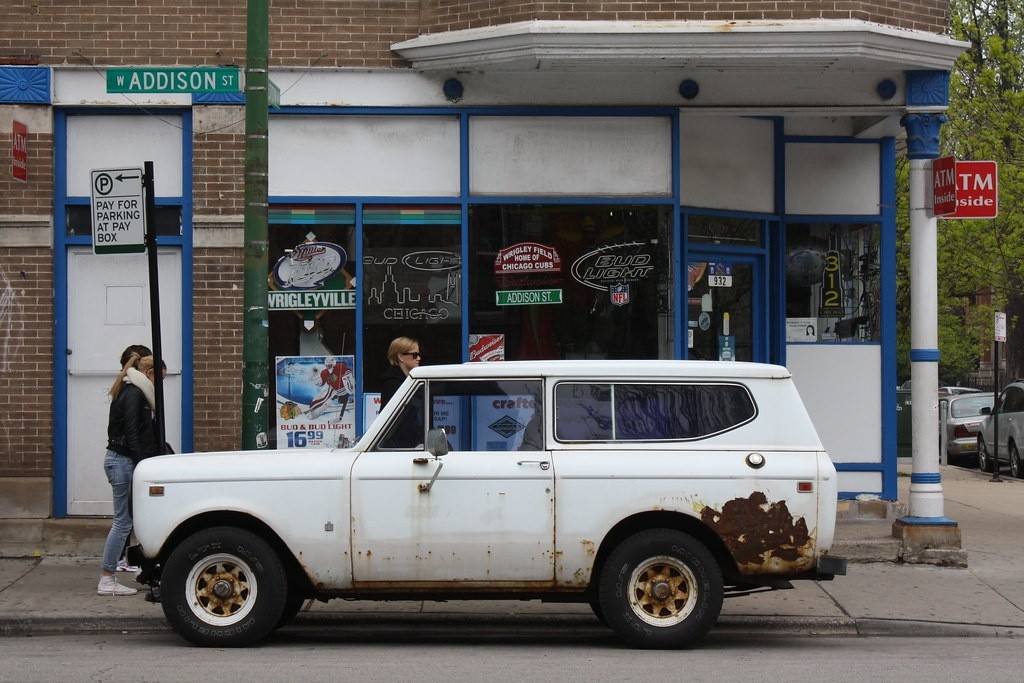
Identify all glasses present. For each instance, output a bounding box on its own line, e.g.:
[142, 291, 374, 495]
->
[402, 351, 421, 359]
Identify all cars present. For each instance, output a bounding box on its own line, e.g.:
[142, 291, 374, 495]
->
[937, 390, 1003, 463]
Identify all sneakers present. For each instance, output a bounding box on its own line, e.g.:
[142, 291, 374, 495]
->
[115, 559, 138, 572]
[97, 578, 138, 596]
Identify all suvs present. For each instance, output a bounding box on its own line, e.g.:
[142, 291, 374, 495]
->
[121, 361, 837, 645]
[978, 377, 1023, 478]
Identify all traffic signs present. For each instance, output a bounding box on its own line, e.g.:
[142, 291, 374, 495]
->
[87, 167, 144, 254]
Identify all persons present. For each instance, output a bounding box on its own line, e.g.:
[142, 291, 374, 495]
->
[82, 351, 167, 596]
[377, 335, 435, 449]
[115, 343, 175, 573]
